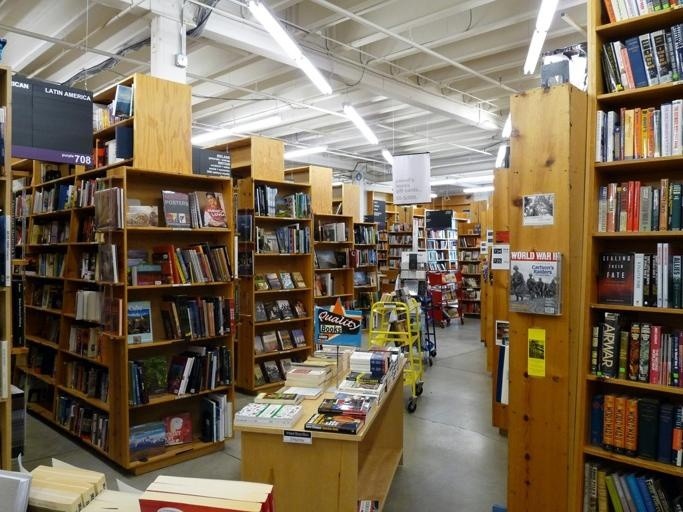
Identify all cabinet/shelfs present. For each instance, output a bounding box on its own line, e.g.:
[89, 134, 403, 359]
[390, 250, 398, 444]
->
[0, 66, 478, 508]
[480, 0, 683, 512]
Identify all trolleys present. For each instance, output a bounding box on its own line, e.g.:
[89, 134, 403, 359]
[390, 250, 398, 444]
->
[366, 292, 437, 413]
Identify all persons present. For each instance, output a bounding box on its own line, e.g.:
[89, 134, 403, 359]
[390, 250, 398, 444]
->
[510, 266, 557, 302]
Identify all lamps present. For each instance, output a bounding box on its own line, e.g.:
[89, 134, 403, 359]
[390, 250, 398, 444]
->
[343, 45, 380, 145]
[246, 1, 335, 96]
